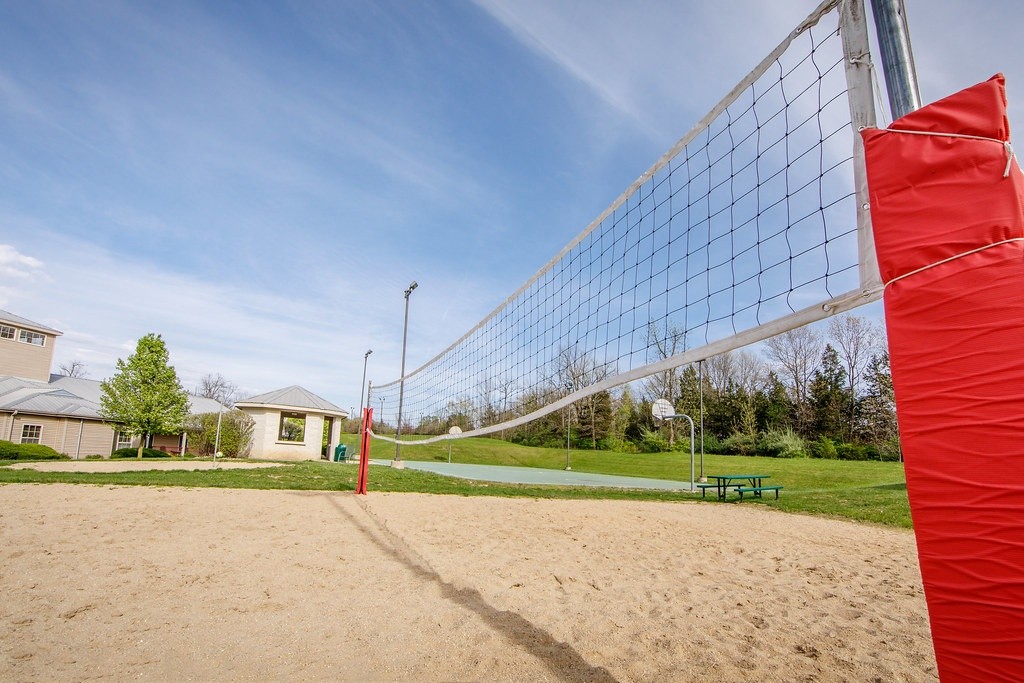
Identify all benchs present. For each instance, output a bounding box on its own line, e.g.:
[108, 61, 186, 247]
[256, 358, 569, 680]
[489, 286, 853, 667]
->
[696, 483, 745, 498]
[734, 486, 783, 503]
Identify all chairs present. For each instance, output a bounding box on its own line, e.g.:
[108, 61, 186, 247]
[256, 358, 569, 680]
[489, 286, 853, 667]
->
[337, 443, 355, 464]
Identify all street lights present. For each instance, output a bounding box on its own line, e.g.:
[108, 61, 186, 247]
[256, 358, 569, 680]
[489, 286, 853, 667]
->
[419, 412, 424, 429]
[563, 381, 574, 470]
[396, 281, 418, 465]
[356, 348, 374, 454]
[695, 358, 707, 481]
[350, 406, 354, 434]
[379, 398, 386, 436]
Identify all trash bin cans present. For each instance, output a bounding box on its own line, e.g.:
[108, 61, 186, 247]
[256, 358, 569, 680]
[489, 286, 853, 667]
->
[334, 443, 346, 461]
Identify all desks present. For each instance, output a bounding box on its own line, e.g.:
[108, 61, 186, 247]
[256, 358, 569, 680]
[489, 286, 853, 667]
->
[707, 475, 771, 503]
